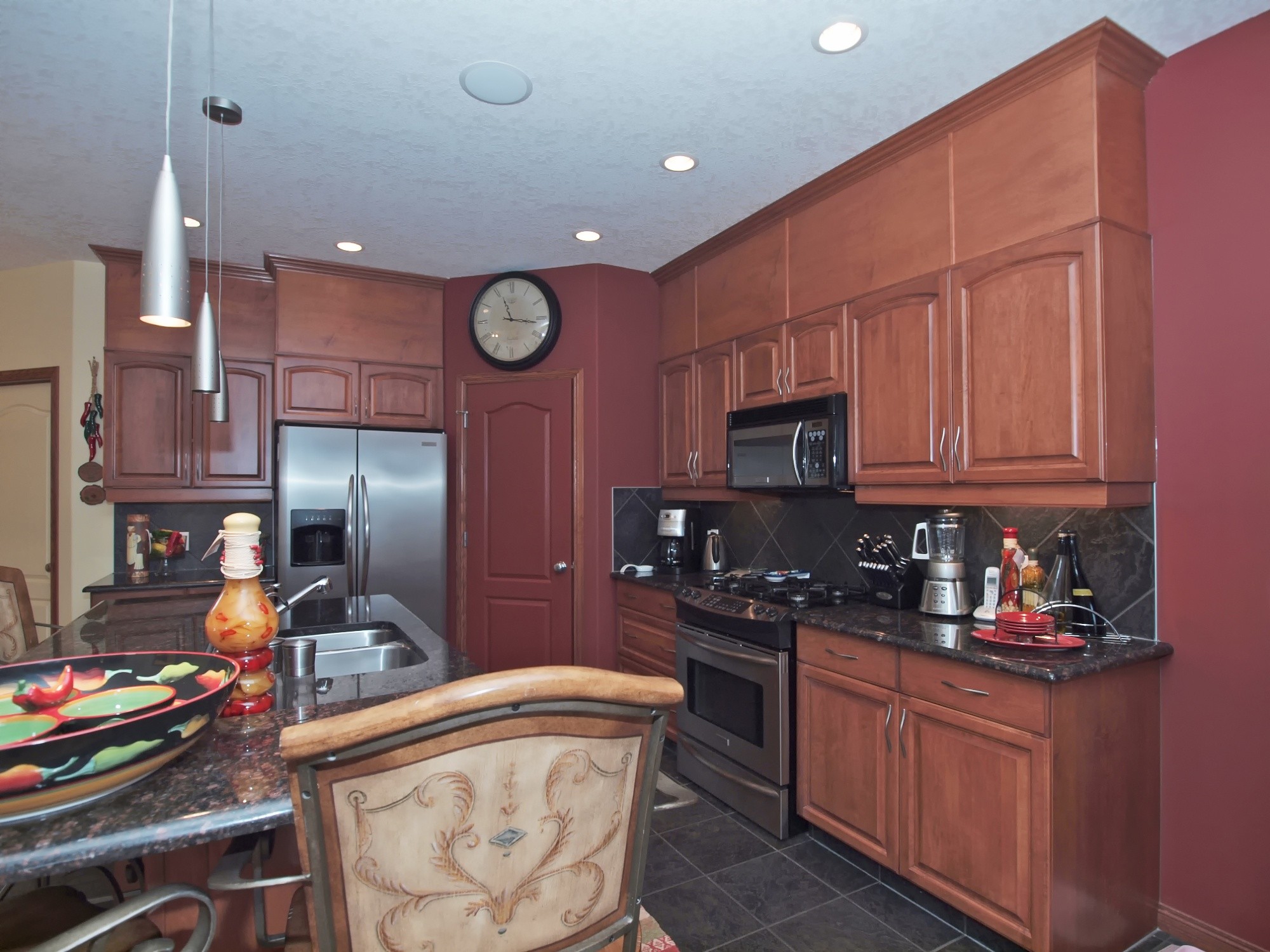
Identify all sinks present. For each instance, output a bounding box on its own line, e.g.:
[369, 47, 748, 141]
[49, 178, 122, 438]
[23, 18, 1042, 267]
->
[274, 618, 422, 682]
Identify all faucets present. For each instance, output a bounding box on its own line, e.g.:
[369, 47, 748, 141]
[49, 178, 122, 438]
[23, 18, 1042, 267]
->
[262, 575, 335, 648]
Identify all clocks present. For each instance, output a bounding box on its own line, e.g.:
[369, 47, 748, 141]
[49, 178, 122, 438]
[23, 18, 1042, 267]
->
[466, 271, 564, 373]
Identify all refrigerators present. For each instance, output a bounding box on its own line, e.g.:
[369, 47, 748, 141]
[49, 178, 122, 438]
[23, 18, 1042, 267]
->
[271, 421, 451, 643]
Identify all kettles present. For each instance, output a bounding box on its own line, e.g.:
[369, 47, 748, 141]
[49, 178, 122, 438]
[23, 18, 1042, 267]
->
[700, 532, 727, 578]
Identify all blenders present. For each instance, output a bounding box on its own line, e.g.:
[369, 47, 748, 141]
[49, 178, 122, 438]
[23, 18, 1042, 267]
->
[910, 507, 975, 616]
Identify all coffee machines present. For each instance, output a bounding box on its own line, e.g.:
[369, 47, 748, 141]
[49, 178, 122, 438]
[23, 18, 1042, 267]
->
[654, 505, 705, 575]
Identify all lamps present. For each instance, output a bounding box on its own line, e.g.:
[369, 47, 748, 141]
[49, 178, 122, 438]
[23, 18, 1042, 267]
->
[139, 0, 234, 426]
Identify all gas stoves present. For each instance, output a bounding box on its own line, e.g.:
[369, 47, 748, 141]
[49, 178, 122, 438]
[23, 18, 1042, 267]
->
[675, 574, 865, 648]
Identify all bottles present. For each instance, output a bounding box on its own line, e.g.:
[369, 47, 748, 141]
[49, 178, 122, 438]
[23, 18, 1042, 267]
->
[1000, 525, 1105, 638]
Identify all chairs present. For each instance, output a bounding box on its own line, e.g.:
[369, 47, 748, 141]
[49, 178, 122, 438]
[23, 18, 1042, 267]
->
[0, 865, 218, 952]
[206, 664, 684, 951]
[0, 564, 63, 666]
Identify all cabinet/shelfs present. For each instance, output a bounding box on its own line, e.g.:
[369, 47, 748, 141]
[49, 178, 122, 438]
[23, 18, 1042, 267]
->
[101, 348, 274, 505]
[848, 221, 1151, 507]
[608, 567, 759, 755]
[274, 354, 445, 433]
[654, 340, 734, 489]
[731, 303, 849, 413]
[799, 606, 1173, 952]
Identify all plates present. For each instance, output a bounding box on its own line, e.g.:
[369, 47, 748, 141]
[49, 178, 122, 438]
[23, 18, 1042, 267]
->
[763, 570, 808, 582]
[970, 612, 1086, 650]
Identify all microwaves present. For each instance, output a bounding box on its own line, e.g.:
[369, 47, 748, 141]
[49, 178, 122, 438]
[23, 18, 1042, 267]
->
[725, 391, 856, 496]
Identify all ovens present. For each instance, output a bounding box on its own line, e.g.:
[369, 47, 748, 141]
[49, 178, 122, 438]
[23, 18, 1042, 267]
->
[670, 622, 808, 842]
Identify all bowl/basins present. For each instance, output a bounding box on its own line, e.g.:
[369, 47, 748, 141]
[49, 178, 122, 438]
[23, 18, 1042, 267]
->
[1, 650, 241, 819]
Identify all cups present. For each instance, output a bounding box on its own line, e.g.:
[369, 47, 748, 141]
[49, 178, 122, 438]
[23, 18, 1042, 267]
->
[268, 637, 283, 675]
[283, 638, 317, 677]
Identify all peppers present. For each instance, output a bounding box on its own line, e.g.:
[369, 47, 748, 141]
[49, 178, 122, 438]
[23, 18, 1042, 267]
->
[0, 652, 241, 802]
